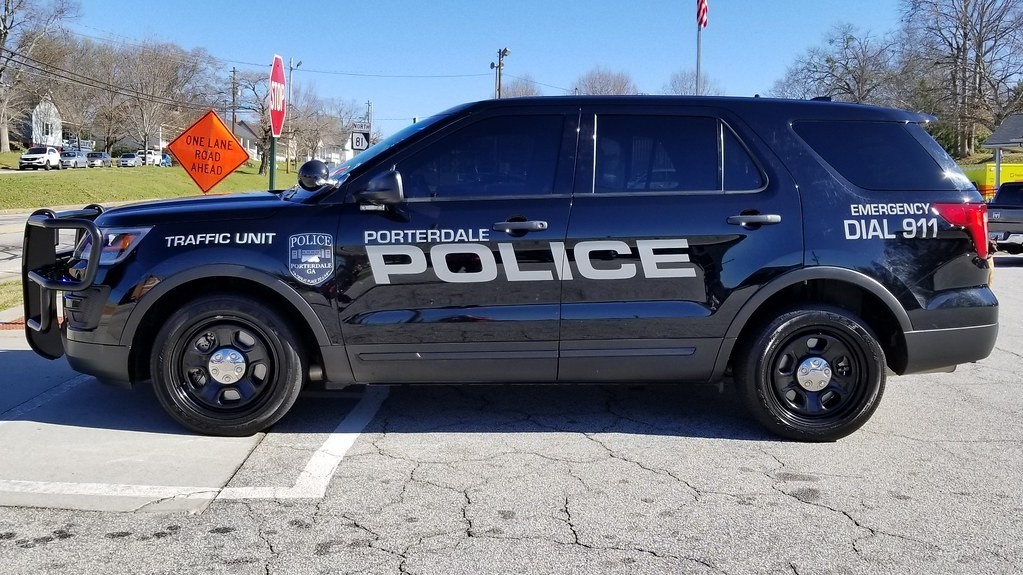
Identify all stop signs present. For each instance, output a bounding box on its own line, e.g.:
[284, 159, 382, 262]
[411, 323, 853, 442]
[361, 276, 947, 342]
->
[269, 54, 286, 138]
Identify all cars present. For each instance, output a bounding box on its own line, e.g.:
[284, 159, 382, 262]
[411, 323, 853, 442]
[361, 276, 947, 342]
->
[86, 152, 113, 167]
[629, 169, 679, 189]
[61, 151, 89, 169]
[116, 153, 142, 167]
[162, 154, 172, 167]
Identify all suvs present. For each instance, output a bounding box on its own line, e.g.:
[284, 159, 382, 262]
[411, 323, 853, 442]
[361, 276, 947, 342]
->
[20, 146, 63, 170]
[20, 92, 1001, 442]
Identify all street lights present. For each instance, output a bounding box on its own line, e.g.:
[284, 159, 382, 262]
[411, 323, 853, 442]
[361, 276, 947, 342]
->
[287, 60, 303, 173]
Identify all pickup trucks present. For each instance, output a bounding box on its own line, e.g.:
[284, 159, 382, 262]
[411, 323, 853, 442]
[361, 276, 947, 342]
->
[984, 180, 1023, 256]
[136, 150, 162, 166]
